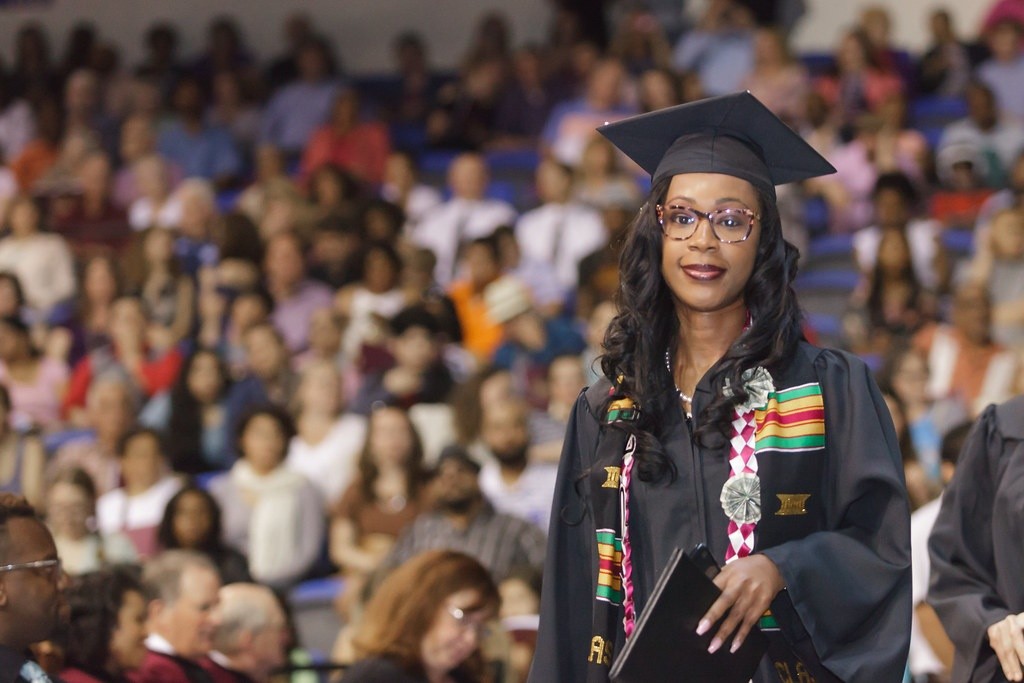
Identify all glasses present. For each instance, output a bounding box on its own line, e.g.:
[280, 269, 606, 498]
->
[656, 204, 763, 244]
[0, 556, 63, 583]
[443, 605, 492, 640]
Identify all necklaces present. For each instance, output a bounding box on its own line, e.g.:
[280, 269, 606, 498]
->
[664, 345, 693, 404]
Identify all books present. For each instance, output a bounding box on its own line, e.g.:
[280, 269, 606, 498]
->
[608, 548, 769, 683]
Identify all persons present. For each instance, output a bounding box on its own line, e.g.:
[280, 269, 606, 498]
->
[522, 88, 913, 682]
[2, 0, 1024, 683]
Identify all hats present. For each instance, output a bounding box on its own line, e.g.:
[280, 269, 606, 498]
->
[595, 90, 838, 202]
